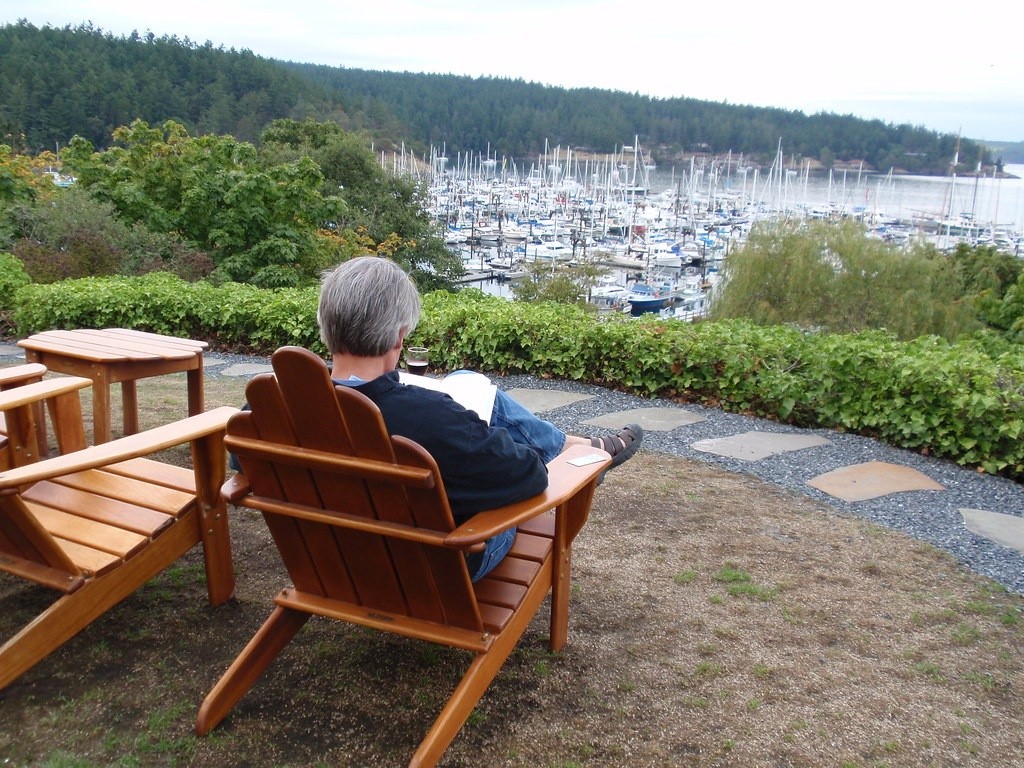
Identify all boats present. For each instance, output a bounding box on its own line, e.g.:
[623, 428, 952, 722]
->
[372, 131, 1024, 321]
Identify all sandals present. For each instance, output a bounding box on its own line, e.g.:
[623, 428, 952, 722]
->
[584, 423, 643, 488]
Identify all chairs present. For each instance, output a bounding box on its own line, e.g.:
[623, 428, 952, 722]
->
[196, 346, 613, 768]
[1, 362, 242, 691]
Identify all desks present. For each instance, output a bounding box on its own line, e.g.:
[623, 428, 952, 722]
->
[17, 327, 209, 457]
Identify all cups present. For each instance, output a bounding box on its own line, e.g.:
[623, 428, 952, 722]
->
[407, 347, 428, 376]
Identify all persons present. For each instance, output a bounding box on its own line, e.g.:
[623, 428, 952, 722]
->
[228, 258, 643, 583]
